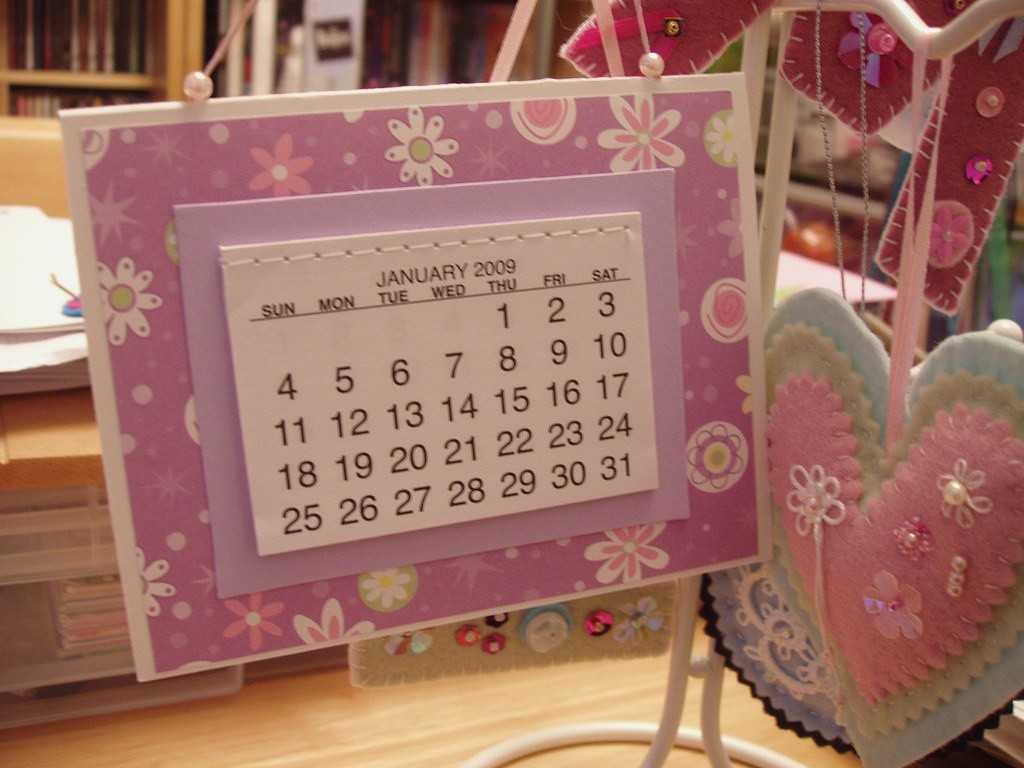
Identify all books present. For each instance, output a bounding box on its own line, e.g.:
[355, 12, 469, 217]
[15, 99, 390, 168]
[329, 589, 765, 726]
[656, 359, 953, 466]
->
[7, 0, 552, 118]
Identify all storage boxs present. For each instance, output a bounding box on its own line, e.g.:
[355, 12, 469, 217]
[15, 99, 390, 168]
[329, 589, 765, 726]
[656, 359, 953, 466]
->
[0, 490, 241, 730]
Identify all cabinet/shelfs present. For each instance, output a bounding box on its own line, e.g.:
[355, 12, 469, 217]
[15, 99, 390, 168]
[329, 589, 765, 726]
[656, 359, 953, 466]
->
[0, 0, 1023, 352]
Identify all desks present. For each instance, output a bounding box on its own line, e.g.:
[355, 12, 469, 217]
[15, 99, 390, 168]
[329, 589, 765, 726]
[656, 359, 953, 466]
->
[0, 388, 1024, 768]
